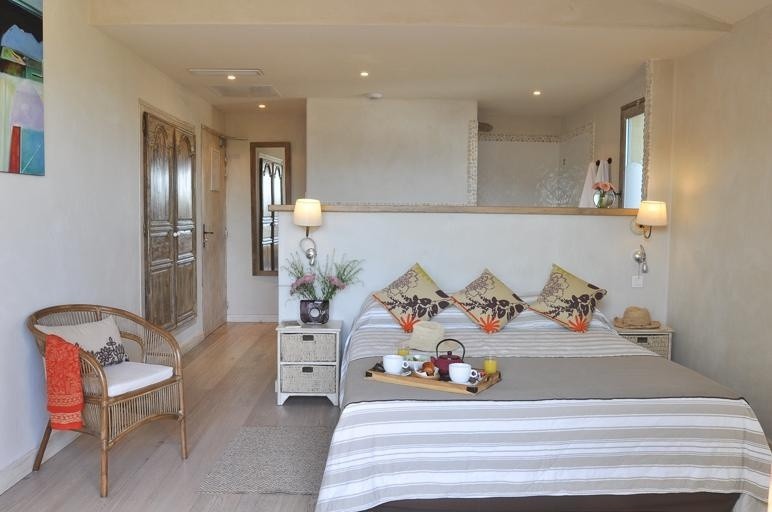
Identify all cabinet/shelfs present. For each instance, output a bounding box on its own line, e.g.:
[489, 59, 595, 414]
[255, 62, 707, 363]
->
[256, 152, 285, 271]
[142, 111, 198, 337]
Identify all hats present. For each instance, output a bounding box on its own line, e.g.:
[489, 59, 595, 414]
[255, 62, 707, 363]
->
[613, 306, 662, 330]
[395, 320, 460, 353]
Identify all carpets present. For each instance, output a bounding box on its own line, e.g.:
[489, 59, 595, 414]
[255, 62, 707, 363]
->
[194, 425, 331, 494]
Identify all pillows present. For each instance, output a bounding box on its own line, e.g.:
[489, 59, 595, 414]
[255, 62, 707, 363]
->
[34, 316, 129, 373]
[528, 264, 608, 333]
[372, 263, 455, 332]
[450, 268, 529, 334]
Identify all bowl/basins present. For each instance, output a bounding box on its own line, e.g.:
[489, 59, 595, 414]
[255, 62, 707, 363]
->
[410, 354, 432, 372]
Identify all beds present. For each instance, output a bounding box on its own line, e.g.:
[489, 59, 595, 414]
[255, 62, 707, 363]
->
[314, 292, 772, 511]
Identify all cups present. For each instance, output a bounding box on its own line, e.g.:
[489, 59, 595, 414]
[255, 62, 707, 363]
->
[382, 354, 409, 375]
[483, 356, 499, 378]
[449, 363, 476, 385]
[398, 347, 409, 356]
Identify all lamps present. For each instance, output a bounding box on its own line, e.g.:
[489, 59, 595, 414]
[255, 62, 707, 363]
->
[630, 201, 667, 239]
[293, 199, 323, 238]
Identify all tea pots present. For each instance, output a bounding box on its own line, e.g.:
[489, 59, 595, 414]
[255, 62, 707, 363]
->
[429, 338, 465, 377]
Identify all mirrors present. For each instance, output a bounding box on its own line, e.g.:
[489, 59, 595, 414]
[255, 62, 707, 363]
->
[250, 141, 291, 277]
[619, 97, 645, 209]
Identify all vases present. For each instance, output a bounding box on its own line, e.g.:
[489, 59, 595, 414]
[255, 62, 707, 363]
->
[593, 190, 615, 208]
[301, 300, 330, 324]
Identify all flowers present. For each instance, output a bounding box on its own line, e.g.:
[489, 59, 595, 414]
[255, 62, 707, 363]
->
[592, 182, 615, 208]
[279, 249, 363, 300]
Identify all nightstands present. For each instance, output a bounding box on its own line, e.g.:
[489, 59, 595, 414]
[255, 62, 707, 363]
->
[275, 320, 341, 406]
[614, 327, 672, 360]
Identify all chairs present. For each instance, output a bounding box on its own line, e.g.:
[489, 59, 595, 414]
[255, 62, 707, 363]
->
[27, 304, 187, 497]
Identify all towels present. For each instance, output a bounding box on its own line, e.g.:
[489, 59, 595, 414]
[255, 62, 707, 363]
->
[579, 162, 597, 207]
[597, 159, 610, 182]
[45, 334, 85, 430]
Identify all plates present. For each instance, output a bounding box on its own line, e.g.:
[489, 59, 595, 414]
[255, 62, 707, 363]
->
[413, 365, 439, 379]
